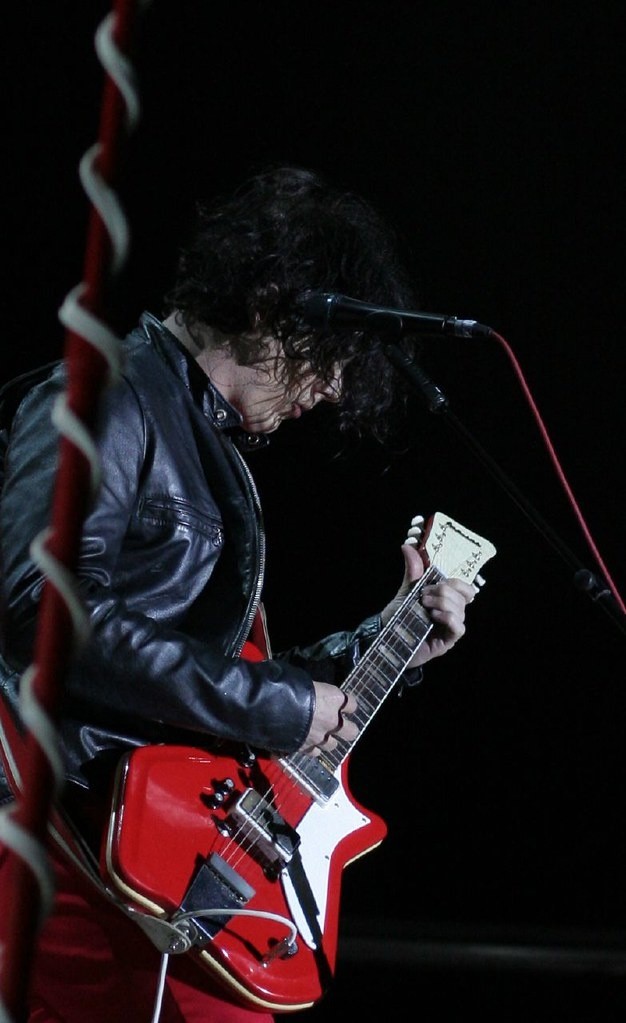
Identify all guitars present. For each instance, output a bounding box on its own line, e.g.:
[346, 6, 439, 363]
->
[97, 509, 500, 1014]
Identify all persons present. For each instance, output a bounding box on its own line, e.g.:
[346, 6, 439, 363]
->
[0, 166, 476, 1023]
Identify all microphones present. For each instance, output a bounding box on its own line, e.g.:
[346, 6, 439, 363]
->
[305, 292, 493, 341]
